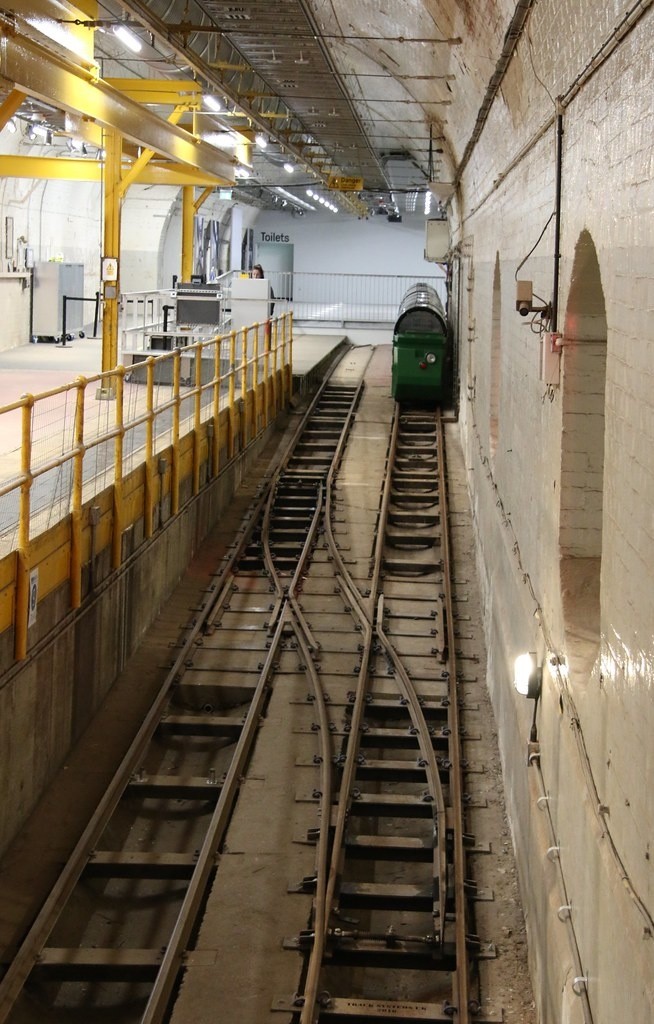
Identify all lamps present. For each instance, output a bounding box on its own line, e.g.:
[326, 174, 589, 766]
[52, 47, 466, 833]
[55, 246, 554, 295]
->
[423, 191, 433, 215]
[112, 24, 141, 52]
[284, 163, 294, 174]
[202, 93, 220, 112]
[306, 189, 338, 213]
[254, 136, 267, 148]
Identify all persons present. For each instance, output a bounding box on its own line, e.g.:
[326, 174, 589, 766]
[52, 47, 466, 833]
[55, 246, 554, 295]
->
[251, 263, 276, 318]
